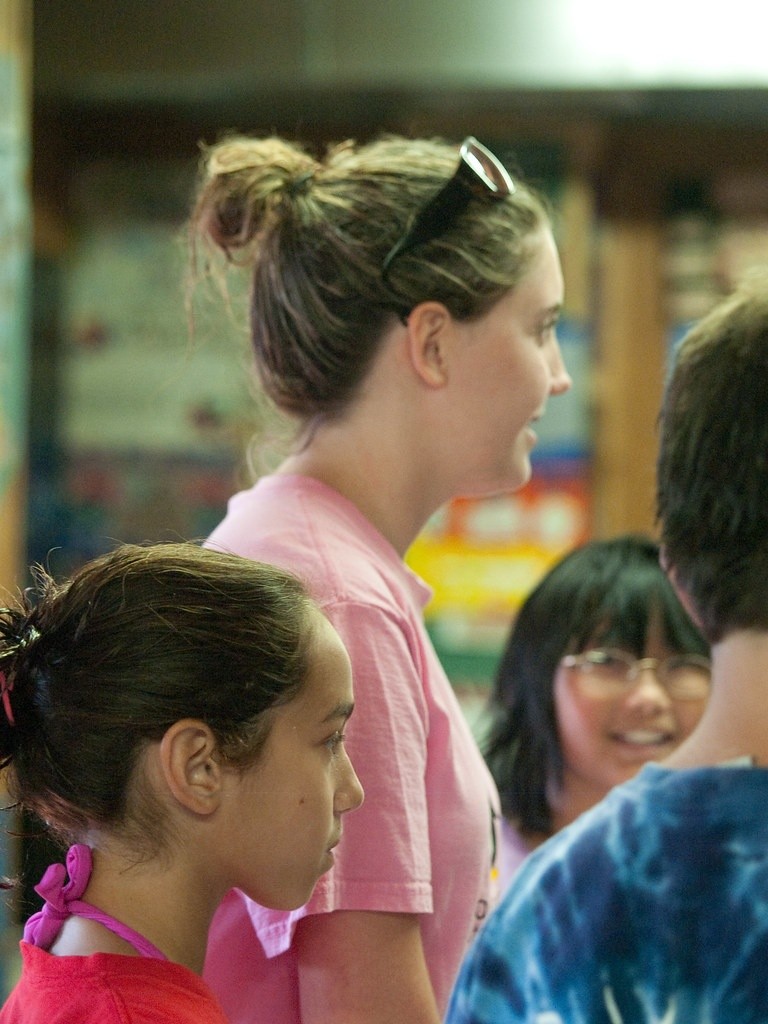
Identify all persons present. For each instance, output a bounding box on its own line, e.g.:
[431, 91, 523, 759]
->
[1, 543, 364, 1024]
[446, 284, 767, 1024]
[197, 132, 569, 1024]
[480, 537, 708, 889]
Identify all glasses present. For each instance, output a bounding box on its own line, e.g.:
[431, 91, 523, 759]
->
[368, 127, 513, 294]
[549, 648, 715, 713]
